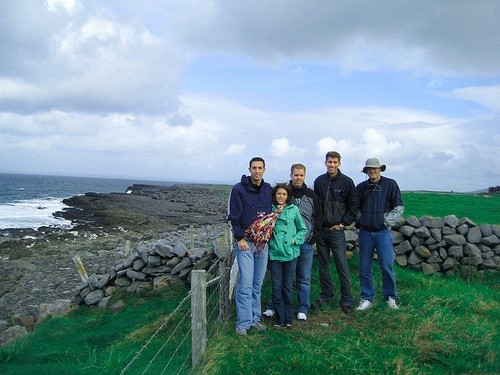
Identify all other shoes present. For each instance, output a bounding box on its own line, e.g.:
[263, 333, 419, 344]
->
[286, 323, 293, 328]
[251, 321, 266, 330]
[311, 298, 327, 310]
[236, 327, 247, 336]
[343, 306, 352, 313]
[273, 324, 283, 330]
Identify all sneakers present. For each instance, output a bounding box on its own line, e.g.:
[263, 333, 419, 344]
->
[297, 313, 306, 321]
[263, 310, 274, 317]
[385, 296, 399, 309]
[356, 298, 372, 310]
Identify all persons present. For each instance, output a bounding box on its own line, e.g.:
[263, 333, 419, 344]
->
[314, 152, 355, 313]
[268, 182, 308, 329]
[227, 157, 273, 336]
[262, 164, 318, 321]
[355, 158, 405, 310]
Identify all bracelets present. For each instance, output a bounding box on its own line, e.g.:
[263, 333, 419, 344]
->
[339, 223, 344, 228]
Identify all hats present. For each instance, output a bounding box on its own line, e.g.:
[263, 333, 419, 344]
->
[363, 158, 386, 172]
